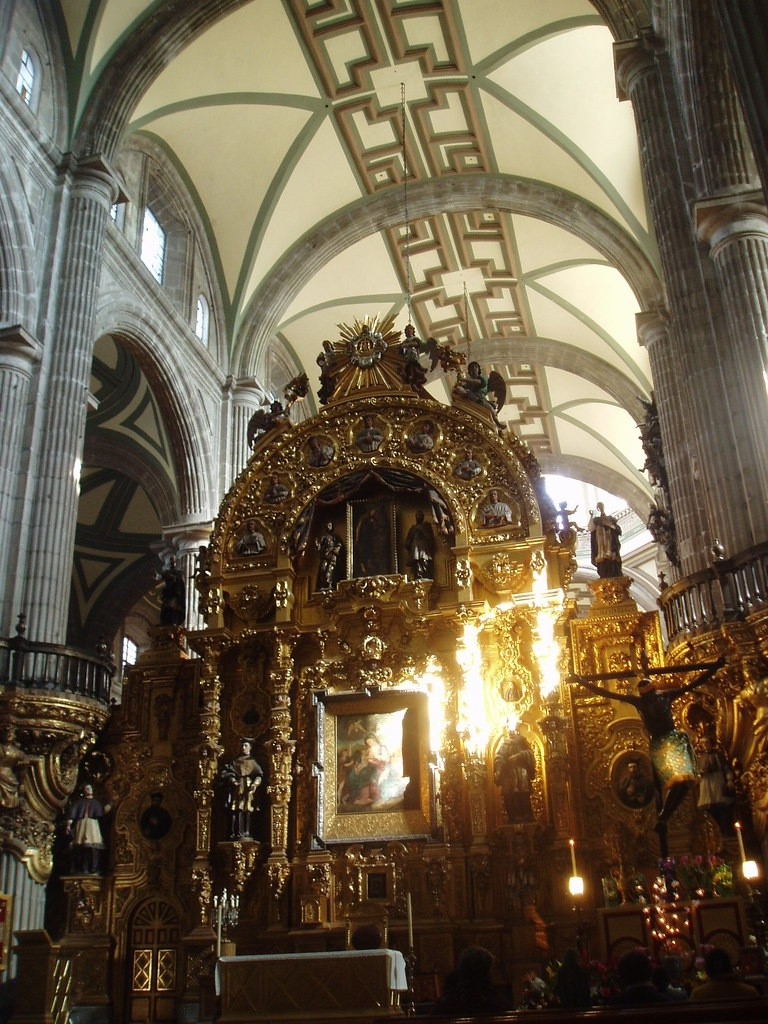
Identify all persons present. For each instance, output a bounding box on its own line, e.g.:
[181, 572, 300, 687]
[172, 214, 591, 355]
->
[402, 324, 427, 387]
[479, 490, 513, 528]
[224, 739, 263, 838]
[308, 440, 333, 465]
[266, 475, 290, 503]
[158, 556, 186, 626]
[697, 736, 737, 838]
[66, 783, 112, 874]
[571, 658, 726, 832]
[238, 521, 268, 556]
[494, 726, 537, 825]
[406, 511, 436, 580]
[455, 448, 482, 480]
[313, 521, 342, 592]
[437, 944, 768, 1024]
[589, 503, 624, 578]
[407, 421, 433, 453]
[355, 416, 385, 453]
[635, 390, 676, 568]
[265, 402, 292, 432]
[456, 362, 507, 430]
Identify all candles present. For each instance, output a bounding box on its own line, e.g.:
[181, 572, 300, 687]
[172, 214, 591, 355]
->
[406, 892, 413, 947]
[569, 839, 577, 877]
[216, 904, 222, 958]
[734, 822, 746, 862]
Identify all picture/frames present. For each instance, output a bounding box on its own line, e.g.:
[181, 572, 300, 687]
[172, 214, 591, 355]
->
[316, 689, 434, 842]
